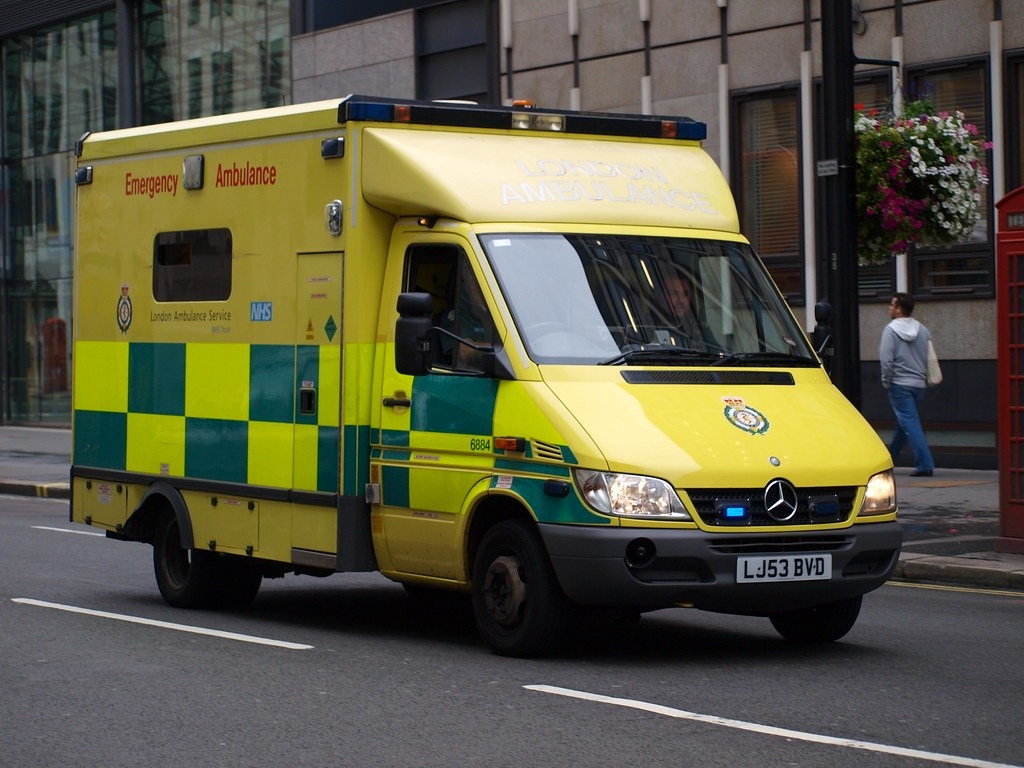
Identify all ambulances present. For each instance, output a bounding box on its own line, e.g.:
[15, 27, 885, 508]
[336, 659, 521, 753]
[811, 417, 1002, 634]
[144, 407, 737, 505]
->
[70, 93, 904, 658]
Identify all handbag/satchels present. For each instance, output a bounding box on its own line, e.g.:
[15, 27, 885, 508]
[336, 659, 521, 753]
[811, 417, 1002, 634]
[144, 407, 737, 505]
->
[926, 339, 943, 386]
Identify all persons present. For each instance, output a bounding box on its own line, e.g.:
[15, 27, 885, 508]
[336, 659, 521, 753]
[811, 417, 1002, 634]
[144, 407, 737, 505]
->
[624, 262, 724, 355]
[414, 260, 502, 369]
[880, 293, 943, 477]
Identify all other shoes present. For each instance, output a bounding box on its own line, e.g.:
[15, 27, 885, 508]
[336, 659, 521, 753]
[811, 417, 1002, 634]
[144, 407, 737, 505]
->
[910, 470, 932, 477]
